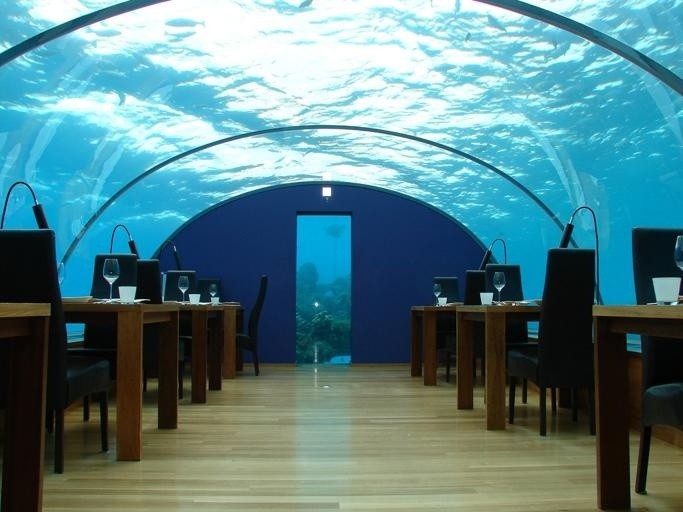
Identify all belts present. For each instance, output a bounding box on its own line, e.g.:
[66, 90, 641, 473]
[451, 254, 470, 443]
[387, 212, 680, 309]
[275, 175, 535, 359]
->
[432, 283, 441, 307]
[493, 272, 506, 305]
[102, 257, 120, 299]
[177, 275, 190, 304]
[208, 283, 218, 298]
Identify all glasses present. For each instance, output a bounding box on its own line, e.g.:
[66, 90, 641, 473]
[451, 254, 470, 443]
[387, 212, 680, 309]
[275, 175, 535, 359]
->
[321, 185, 332, 203]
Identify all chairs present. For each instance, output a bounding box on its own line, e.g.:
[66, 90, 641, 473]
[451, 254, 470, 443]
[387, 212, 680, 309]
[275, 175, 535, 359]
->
[509, 250, 595, 435]
[65, 254, 142, 421]
[2, 229, 109, 475]
[133, 261, 240, 404]
[410, 265, 543, 431]
[237, 275, 268, 377]
[632, 227, 683, 494]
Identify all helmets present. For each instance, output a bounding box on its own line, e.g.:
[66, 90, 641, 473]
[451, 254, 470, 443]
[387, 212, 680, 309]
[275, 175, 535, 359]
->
[60, 296, 93, 303]
[111, 297, 150, 303]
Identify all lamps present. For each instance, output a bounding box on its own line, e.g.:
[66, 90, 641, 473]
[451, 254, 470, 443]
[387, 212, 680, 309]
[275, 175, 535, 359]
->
[321, 185, 332, 203]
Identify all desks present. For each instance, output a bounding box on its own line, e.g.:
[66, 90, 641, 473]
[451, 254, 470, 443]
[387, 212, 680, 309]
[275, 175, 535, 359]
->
[0, 301, 52, 510]
[593, 304, 682, 510]
[61, 299, 180, 461]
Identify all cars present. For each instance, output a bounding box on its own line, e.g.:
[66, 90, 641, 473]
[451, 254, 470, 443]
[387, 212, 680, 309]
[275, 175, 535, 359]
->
[188, 293, 201, 304]
[53, 261, 65, 287]
[651, 276, 681, 306]
[478, 292, 493, 305]
[118, 285, 137, 304]
[210, 297, 220, 305]
[438, 297, 447, 306]
[674, 236, 683, 272]
